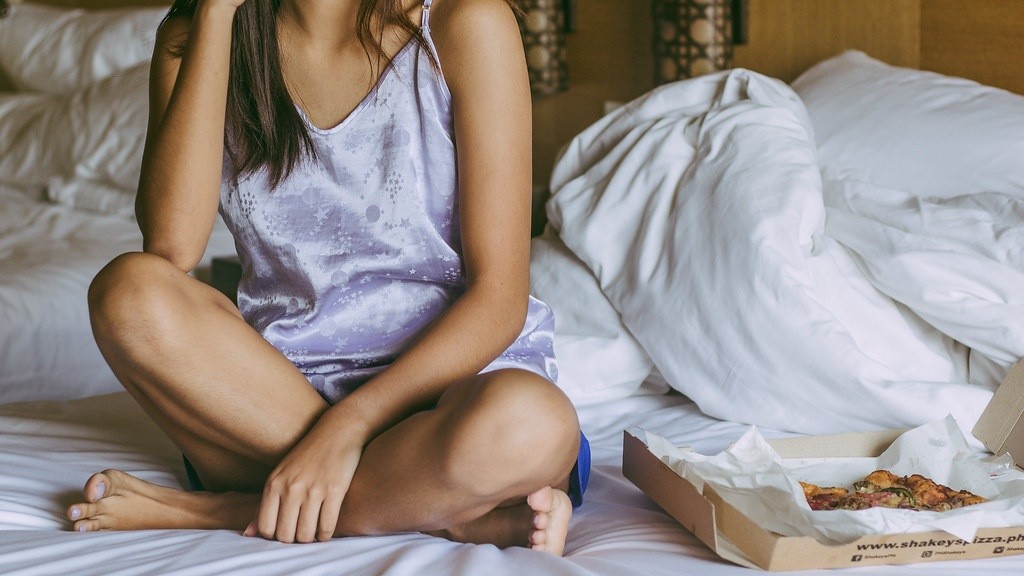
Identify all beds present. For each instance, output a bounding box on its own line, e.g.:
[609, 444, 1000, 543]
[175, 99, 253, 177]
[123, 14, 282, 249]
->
[1, 181, 239, 401]
[0, 391, 1023, 575]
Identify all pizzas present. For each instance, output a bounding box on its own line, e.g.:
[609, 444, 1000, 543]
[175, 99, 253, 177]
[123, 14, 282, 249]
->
[796, 470, 990, 510]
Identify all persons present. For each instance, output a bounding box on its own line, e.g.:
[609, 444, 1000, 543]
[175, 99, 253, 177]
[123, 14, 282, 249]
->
[66, 0, 593, 557]
